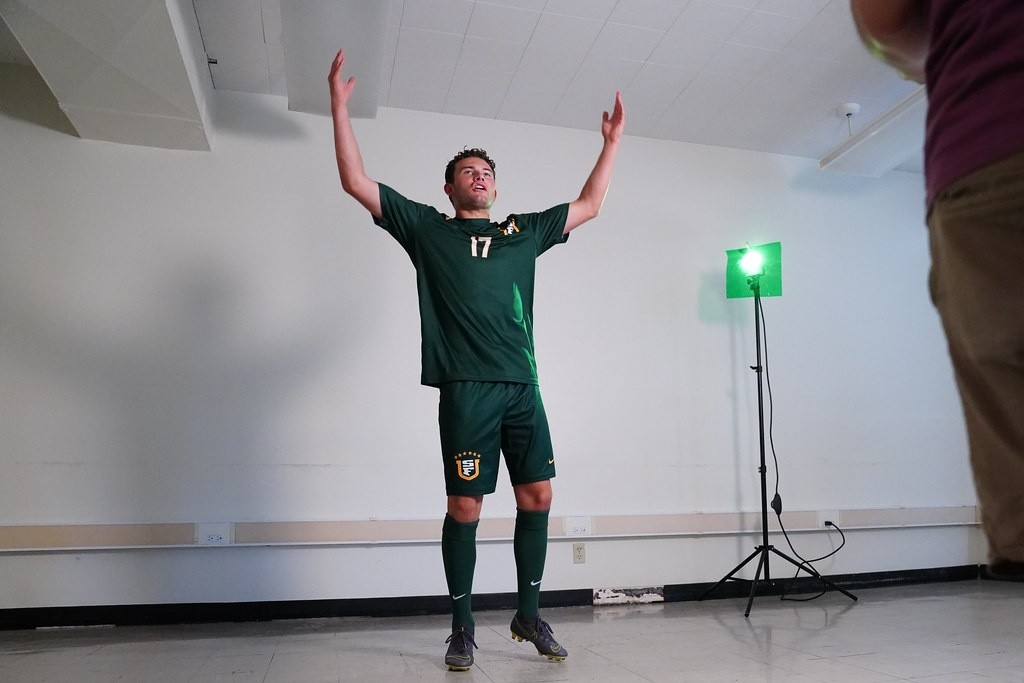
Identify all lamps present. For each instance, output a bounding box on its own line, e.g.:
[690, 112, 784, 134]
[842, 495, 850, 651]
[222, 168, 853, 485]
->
[819, 85, 926, 168]
[699, 251, 859, 616]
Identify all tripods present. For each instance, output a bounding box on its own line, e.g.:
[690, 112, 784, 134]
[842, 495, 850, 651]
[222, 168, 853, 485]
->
[698, 282, 858, 618]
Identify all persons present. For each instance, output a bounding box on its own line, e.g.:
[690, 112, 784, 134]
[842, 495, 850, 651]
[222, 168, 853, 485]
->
[327, 47, 626, 672]
[849, 0, 1023, 580]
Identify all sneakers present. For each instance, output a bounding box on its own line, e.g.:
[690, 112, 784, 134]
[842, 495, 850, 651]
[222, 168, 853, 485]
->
[445, 625, 478, 670]
[510, 614, 568, 661]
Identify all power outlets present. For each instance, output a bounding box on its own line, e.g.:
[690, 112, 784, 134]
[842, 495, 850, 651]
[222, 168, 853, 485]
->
[573, 542, 585, 564]
[818, 510, 839, 528]
[194, 523, 236, 545]
[563, 516, 591, 536]
